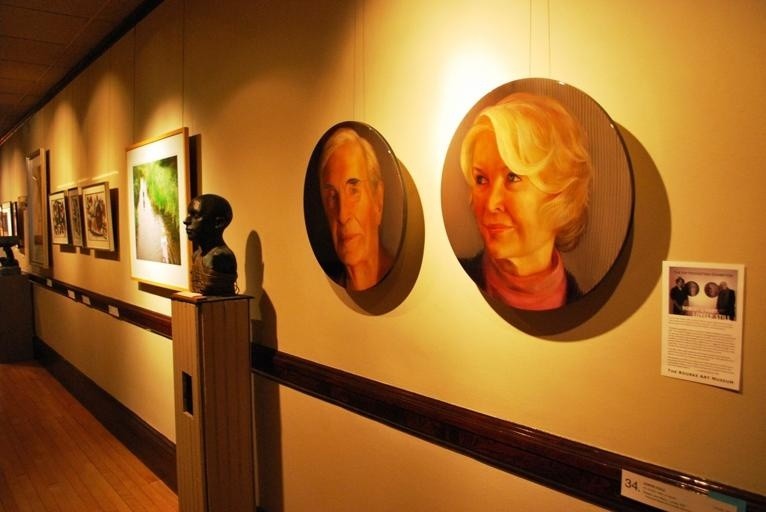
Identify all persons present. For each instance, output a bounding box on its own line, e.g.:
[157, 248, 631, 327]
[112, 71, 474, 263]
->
[319, 128, 395, 292]
[456, 93, 595, 311]
[183, 194, 238, 297]
[671, 277, 689, 315]
[711, 281, 735, 320]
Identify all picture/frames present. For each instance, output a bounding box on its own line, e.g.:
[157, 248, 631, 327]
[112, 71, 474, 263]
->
[124, 123, 194, 294]
[65, 186, 84, 248]
[24, 145, 50, 270]
[0, 195, 23, 249]
[81, 177, 114, 253]
[47, 187, 70, 245]
